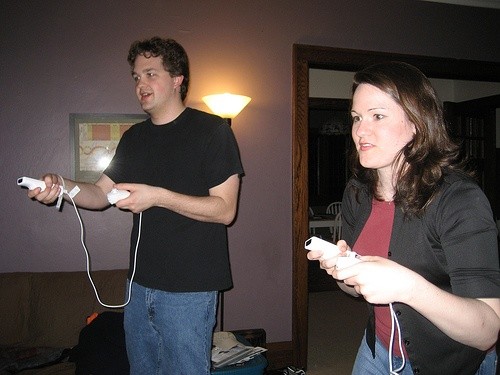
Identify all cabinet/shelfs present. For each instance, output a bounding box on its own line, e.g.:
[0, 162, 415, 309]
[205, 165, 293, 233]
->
[308, 94, 500, 224]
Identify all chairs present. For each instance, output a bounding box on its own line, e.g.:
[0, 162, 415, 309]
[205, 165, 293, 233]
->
[308, 202, 343, 280]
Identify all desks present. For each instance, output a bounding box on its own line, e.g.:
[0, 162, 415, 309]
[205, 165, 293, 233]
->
[309, 214, 344, 244]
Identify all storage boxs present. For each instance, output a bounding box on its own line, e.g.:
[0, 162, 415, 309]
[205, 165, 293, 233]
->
[210, 333, 269, 375]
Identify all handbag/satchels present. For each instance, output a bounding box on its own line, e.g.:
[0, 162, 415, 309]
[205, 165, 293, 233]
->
[69, 305, 131, 375]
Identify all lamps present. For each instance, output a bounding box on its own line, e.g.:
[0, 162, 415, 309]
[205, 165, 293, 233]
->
[201, 93, 252, 129]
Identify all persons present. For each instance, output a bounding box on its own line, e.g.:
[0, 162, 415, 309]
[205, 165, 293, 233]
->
[306, 60, 500, 375]
[26, 36, 246, 375]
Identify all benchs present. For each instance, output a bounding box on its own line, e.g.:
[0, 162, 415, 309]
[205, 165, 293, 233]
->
[0, 268, 131, 375]
